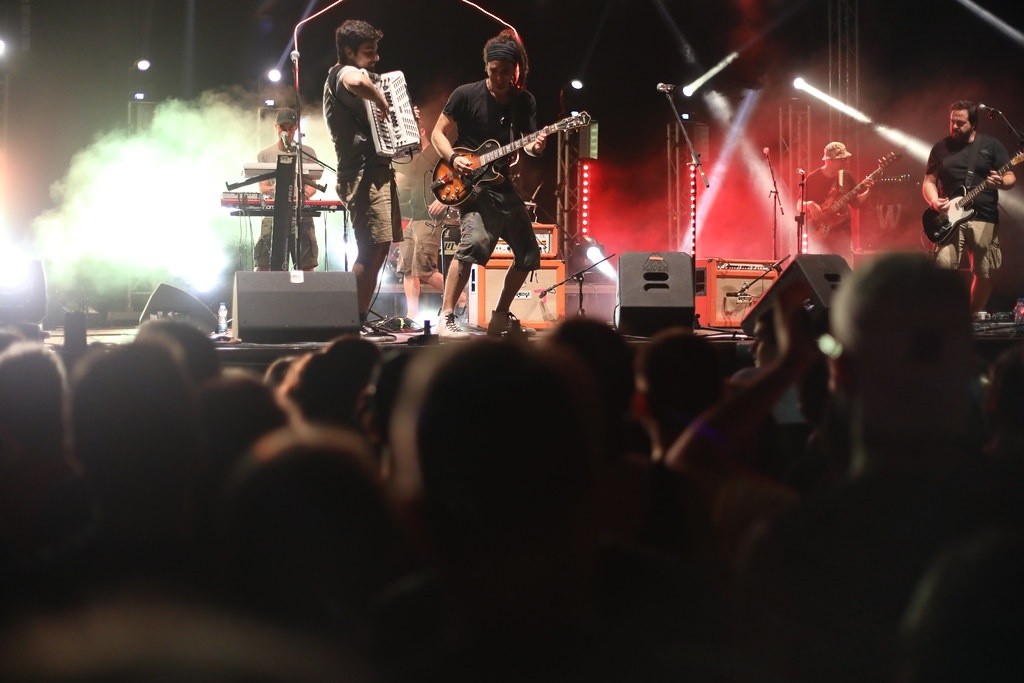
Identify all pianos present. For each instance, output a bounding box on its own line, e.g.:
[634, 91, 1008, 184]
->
[221, 191, 346, 217]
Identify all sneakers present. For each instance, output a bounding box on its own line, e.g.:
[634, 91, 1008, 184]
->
[359, 324, 397, 342]
[436, 309, 471, 339]
[487, 310, 537, 336]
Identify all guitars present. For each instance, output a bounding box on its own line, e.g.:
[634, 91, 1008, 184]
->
[920, 144, 1024, 248]
[804, 151, 903, 242]
[431, 110, 591, 208]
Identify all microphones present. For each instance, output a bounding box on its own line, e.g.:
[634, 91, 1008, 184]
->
[539, 291, 546, 298]
[290, 50, 300, 61]
[763, 148, 769, 153]
[796, 168, 805, 175]
[281, 130, 290, 149]
[657, 83, 677, 92]
[979, 105, 1000, 114]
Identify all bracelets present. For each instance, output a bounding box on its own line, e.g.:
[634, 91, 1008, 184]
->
[304, 189, 310, 200]
[532, 143, 542, 157]
[450, 154, 457, 170]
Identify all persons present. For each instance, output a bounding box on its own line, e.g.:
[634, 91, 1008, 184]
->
[795, 141, 875, 271]
[254, 108, 325, 272]
[0, 250, 1024, 683]
[921, 101, 1016, 321]
[431, 31, 548, 339]
[323, 19, 421, 343]
[396, 120, 467, 323]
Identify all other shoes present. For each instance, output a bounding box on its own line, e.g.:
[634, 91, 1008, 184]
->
[456, 304, 467, 316]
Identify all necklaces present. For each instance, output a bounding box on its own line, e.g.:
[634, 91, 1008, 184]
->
[488, 79, 498, 97]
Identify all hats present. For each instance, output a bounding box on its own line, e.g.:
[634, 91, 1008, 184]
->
[276, 108, 297, 125]
[822, 141, 852, 161]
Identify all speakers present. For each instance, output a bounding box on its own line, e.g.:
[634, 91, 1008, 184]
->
[130, 260, 206, 314]
[469, 258, 565, 329]
[740, 254, 851, 338]
[232, 270, 360, 343]
[615, 251, 694, 335]
[708, 269, 778, 329]
[693, 258, 713, 330]
[139, 282, 219, 336]
